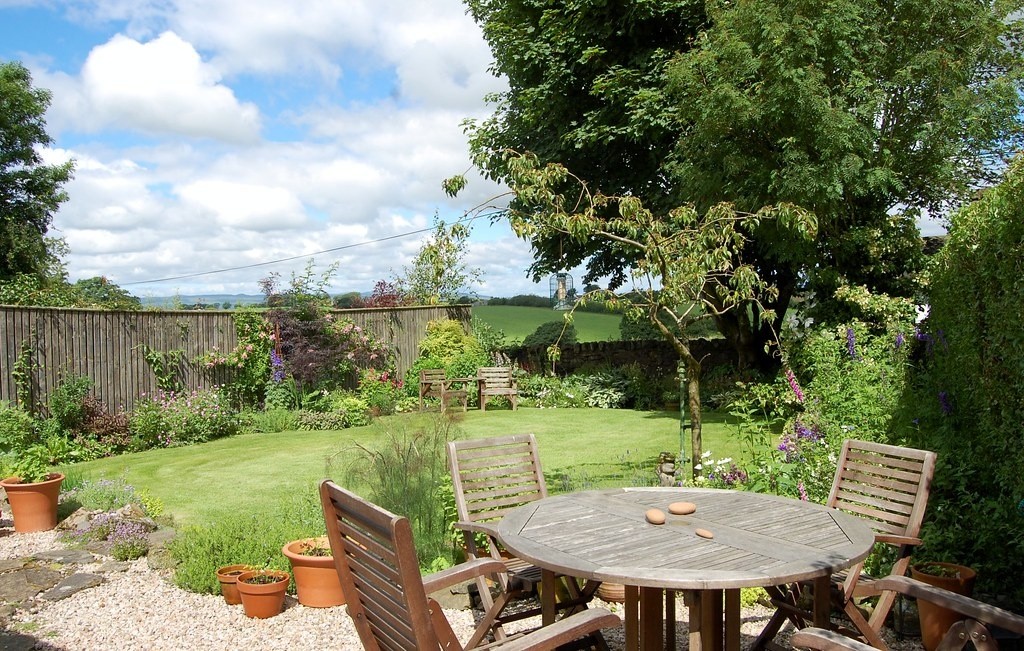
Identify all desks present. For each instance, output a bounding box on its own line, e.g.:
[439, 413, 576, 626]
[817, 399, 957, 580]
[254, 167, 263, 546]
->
[498, 485, 875, 651]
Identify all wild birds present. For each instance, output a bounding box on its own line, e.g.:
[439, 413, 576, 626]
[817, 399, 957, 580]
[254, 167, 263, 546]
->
[558, 277, 566, 308]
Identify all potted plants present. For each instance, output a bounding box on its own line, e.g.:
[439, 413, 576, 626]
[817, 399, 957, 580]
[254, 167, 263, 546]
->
[284, 536, 367, 607]
[0, 399, 73, 533]
[236, 560, 290, 619]
[911, 560, 975, 651]
[215, 564, 258, 605]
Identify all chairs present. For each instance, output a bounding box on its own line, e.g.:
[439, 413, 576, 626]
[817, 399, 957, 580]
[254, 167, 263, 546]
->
[476, 367, 518, 411]
[749, 438, 938, 650]
[318, 478, 621, 651]
[419, 369, 474, 413]
[446, 432, 610, 650]
[790, 573, 1024, 651]
